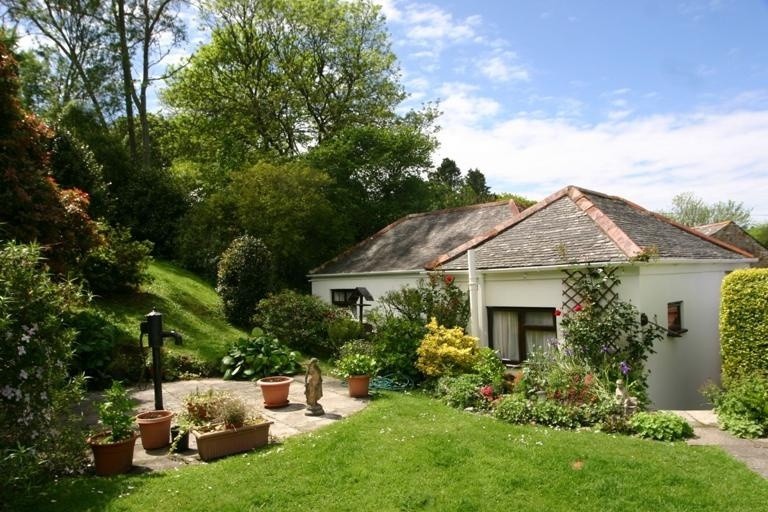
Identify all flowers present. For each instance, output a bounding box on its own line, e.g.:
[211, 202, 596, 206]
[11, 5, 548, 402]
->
[480, 304, 631, 407]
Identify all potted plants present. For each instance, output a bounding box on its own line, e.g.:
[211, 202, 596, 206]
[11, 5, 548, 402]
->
[168, 386, 274, 462]
[87, 377, 139, 475]
[328, 338, 401, 399]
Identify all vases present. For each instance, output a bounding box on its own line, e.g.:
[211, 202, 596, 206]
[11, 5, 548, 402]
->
[536, 391, 548, 405]
[135, 410, 175, 449]
[256, 376, 294, 408]
